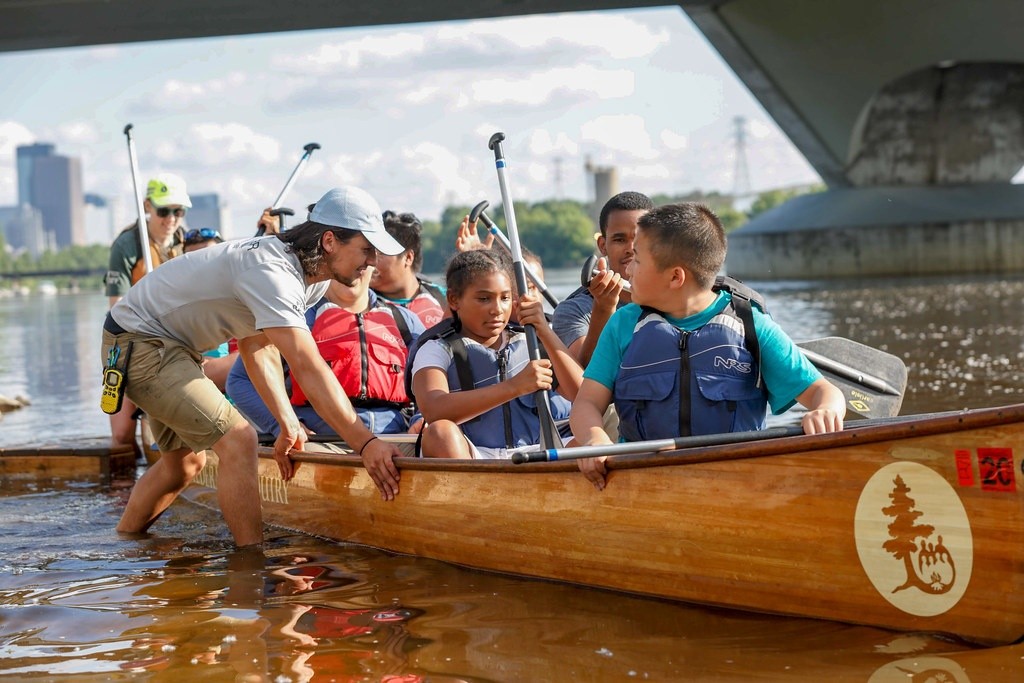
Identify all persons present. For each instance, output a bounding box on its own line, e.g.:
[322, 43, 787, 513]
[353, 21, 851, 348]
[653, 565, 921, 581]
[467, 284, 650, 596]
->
[456, 215, 544, 330]
[183, 207, 280, 390]
[404, 250, 585, 460]
[101, 173, 192, 466]
[101, 186, 404, 547]
[226, 265, 427, 454]
[552, 191, 656, 370]
[369, 210, 453, 330]
[569, 201, 847, 490]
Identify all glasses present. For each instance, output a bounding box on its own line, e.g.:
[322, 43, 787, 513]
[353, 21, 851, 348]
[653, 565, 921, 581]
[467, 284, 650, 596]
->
[148, 200, 185, 217]
[183, 228, 225, 242]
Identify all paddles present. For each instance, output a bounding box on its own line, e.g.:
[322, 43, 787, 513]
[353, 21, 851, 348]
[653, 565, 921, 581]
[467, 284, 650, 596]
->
[573, 254, 910, 422]
[256, 137, 320, 238]
[487, 129, 558, 456]
[468, 199, 563, 315]
[505, 403, 1000, 467]
[265, 206, 293, 237]
[123, 120, 156, 277]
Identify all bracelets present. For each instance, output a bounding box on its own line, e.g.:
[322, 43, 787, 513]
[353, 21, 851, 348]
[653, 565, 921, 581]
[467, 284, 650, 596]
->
[360, 437, 377, 455]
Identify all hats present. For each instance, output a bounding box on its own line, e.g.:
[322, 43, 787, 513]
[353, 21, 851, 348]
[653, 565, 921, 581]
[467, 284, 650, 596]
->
[146, 175, 192, 208]
[307, 185, 405, 256]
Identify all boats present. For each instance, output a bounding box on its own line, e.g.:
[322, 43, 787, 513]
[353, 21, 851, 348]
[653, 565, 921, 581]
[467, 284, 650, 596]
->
[141, 400, 1024, 649]
